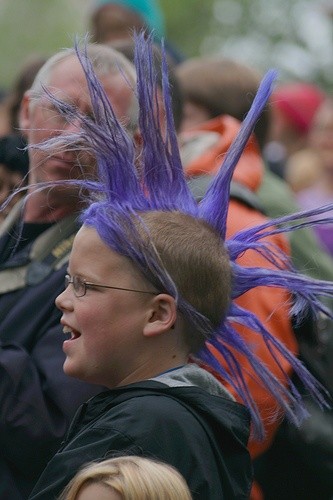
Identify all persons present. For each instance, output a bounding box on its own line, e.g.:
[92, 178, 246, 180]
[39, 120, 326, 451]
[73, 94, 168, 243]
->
[0, 43, 143, 500]
[58, 456, 192, 500]
[0, 0, 333, 500]
[0, 27, 332, 500]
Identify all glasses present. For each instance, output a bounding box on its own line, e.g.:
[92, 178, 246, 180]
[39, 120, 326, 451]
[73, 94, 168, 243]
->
[64, 274, 158, 300]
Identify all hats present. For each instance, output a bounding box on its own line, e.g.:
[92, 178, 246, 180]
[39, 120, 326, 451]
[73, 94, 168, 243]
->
[271, 84, 323, 137]
